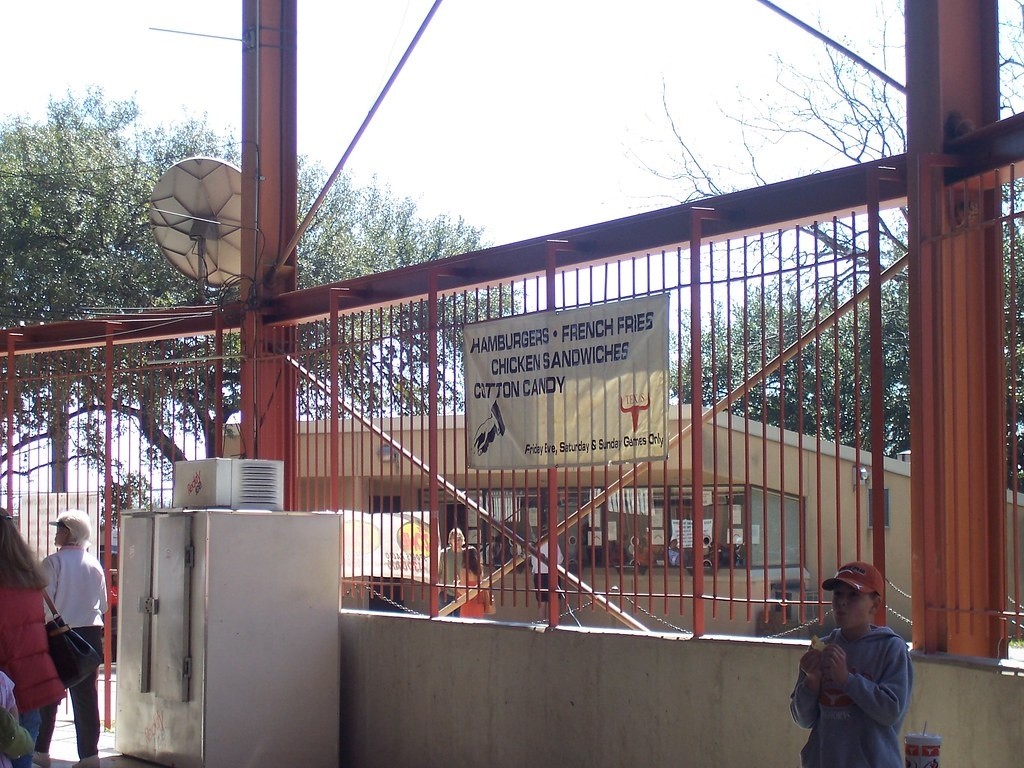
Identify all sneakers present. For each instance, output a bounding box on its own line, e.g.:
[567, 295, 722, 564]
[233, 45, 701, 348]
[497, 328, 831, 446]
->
[31, 752, 51, 767]
[72, 755, 101, 768]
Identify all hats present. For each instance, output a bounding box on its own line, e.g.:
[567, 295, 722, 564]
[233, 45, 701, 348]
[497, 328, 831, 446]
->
[48, 510, 91, 544]
[822, 562, 884, 596]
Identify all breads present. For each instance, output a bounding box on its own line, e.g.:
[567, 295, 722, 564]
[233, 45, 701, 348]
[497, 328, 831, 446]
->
[811, 635, 828, 652]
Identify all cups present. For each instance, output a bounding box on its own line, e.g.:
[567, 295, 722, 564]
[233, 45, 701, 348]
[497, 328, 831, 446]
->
[904, 733, 942, 768]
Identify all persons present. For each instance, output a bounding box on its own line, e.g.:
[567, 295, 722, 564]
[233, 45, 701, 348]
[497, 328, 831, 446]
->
[0, 507, 109, 768]
[437, 527, 487, 619]
[532, 525, 564, 626]
[789, 562, 914, 768]
[667, 537, 683, 567]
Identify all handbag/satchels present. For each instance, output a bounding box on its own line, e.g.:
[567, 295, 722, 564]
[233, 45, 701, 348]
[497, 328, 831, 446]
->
[483, 590, 497, 615]
[0, 706, 34, 759]
[45, 616, 102, 688]
